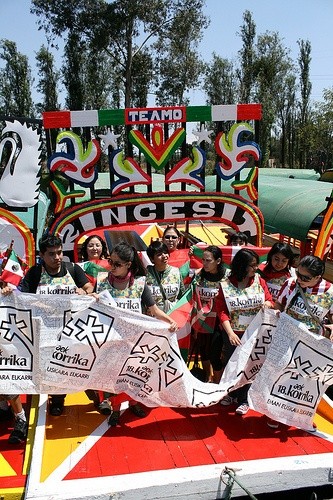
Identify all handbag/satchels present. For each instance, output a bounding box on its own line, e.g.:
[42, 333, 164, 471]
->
[190, 365, 210, 383]
[152, 266, 178, 314]
[322, 324, 333, 343]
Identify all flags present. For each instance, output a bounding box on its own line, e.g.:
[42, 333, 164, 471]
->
[0, 249, 24, 287]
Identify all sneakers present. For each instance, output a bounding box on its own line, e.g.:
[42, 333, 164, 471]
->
[0, 408, 13, 422]
[236, 404, 249, 414]
[220, 396, 234, 405]
[9, 417, 28, 444]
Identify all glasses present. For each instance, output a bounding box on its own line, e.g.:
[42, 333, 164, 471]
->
[108, 259, 128, 268]
[248, 264, 259, 270]
[164, 235, 178, 239]
[296, 271, 314, 282]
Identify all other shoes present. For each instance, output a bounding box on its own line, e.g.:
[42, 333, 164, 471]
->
[267, 417, 279, 428]
[99, 399, 111, 415]
[129, 404, 146, 417]
[50, 397, 64, 416]
[309, 422, 317, 432]
[108, 410, 121, 427]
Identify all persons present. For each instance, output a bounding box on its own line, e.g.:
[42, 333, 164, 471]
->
[0, 226, 333, 446]
